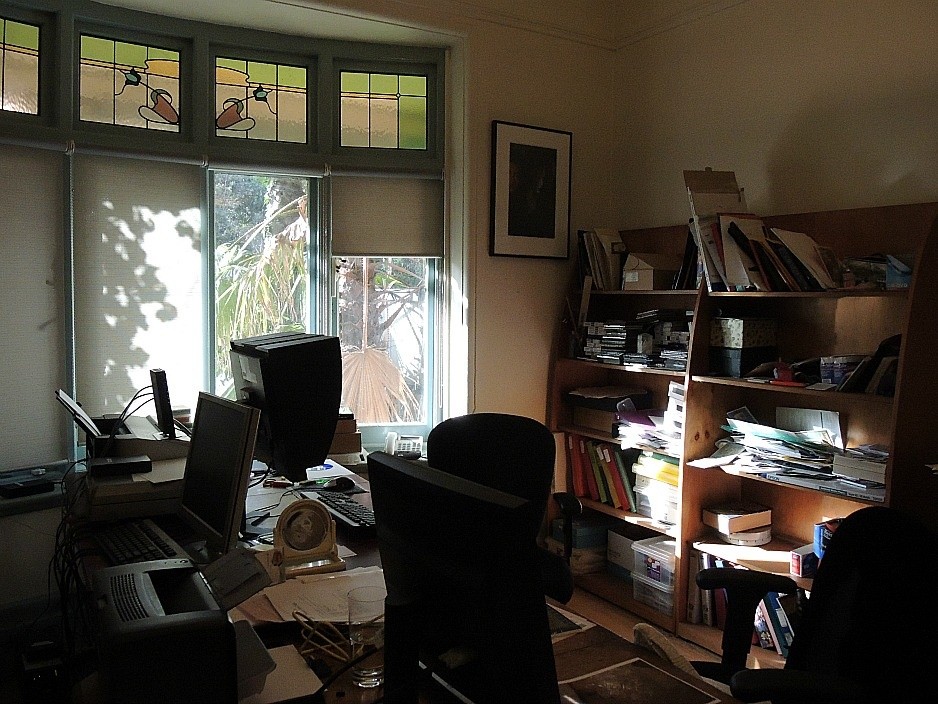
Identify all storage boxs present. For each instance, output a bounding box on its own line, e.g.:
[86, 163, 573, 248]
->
[700, 498, 771, 534]
[544, 516, 676, 617]
[710, 315, 792, 379]
[623, 252, 680, 290]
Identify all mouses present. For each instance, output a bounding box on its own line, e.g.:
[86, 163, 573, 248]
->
[322, 476, 354, 490]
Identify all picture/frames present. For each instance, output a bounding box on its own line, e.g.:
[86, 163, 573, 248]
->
[488, 119, 573, 261]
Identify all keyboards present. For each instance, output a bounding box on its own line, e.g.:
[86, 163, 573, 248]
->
[300, 490, 374, 532]
[90, 519, 194, 563]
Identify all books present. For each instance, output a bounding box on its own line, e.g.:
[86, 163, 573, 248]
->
[566, 436, 640, 515]
[683, 502, 806, 658]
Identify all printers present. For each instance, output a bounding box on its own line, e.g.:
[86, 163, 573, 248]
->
[79, 544, 271, 703]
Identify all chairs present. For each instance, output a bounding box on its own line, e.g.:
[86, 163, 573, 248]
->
[427, 413, 587, 607]
[689, 501, 938, 704]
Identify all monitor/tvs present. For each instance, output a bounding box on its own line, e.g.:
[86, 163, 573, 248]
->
[367, 450, 561, 702]
[177, 392, 261, 554]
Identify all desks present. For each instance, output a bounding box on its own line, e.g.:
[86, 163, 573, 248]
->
[0, 468, 106, 704]
[227, 455, 742, 704]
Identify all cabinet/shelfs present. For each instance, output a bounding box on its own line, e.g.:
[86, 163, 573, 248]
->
[540, 199, 938, 672]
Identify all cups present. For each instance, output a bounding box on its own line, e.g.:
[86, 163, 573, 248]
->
[347, 585, 387, 688]
